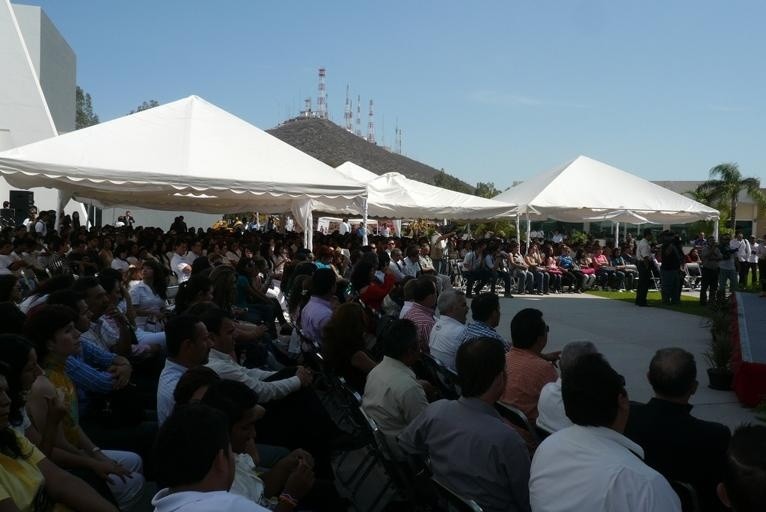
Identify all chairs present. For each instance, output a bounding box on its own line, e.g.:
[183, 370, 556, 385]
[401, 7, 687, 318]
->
[429, 474, 484, 512]
[443, 366, 461, 399]
[449, 257, 466, 293]
[419, 350, 446, 399]
[358, 298, 382, 321]
[335, 372, 376, 486]
[293, 320, 344, 432]
[670, 481, 698, 512]
[535, 417, 556, 442]
[494, 401, 532, 434]
[686, 262, 703, 292]
[350, 406, 428, 511]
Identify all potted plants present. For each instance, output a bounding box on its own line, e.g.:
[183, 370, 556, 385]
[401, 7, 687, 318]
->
[701, 302, 737, 391]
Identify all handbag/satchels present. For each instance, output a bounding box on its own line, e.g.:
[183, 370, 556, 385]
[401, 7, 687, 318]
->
[34, 463, 121, 511]
[146, 313, 169, 332]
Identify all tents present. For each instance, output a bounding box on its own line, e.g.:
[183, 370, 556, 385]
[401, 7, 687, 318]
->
[0, 95, 720, 253]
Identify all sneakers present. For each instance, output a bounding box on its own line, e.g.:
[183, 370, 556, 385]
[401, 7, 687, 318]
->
[467, 285, 634, 298]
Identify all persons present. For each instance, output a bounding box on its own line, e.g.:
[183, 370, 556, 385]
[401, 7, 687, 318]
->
[2, 201, 765, 512]
